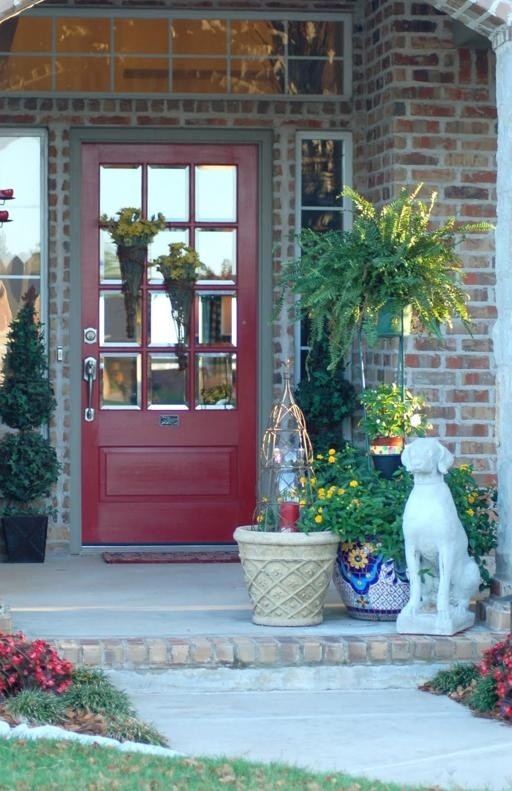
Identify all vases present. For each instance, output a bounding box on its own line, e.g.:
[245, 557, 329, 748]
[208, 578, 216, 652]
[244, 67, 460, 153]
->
[232, 522, 498, 628]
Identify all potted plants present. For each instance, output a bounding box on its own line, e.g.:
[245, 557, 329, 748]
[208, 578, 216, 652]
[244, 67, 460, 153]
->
[358, 383, 435, 484]
[0, 282, 65, 563]
[284, 372, 360, 457]
[273, 180, 496, 382]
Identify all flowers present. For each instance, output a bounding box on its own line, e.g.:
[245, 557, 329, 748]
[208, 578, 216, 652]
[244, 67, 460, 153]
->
[255, 437, 498, 593]
[98, 206, 208, 323]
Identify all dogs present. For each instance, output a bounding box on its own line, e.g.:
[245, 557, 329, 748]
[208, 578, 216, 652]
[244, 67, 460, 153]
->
[401, 437, 481, 619]
